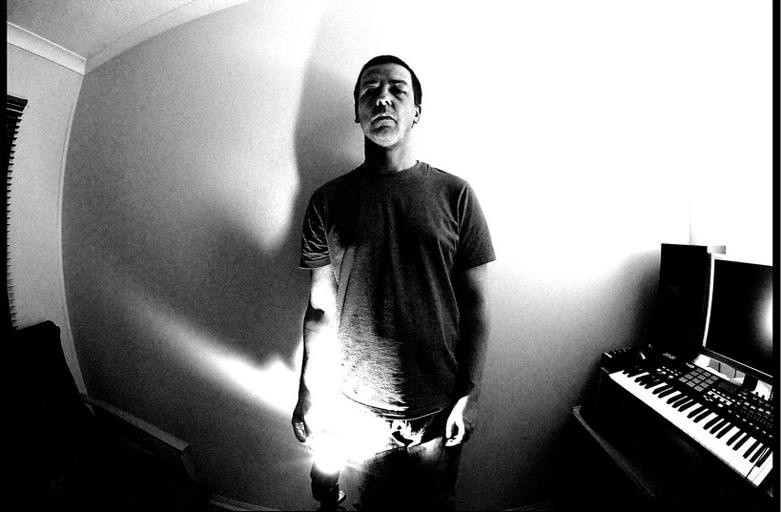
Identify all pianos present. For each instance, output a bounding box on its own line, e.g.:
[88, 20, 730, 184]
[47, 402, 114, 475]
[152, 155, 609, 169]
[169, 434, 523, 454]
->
[583, 340, 780, 506]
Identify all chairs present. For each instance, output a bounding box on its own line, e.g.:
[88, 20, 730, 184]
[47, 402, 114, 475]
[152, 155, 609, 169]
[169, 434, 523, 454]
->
[1, 319, 219, 512]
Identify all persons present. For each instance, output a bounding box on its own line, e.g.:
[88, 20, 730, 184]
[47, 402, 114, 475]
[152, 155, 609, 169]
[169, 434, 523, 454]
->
[293, 55, 500, 510]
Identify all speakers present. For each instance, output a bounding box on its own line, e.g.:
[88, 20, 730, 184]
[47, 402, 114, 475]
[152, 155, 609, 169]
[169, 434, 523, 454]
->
[656, 244, 710, 360]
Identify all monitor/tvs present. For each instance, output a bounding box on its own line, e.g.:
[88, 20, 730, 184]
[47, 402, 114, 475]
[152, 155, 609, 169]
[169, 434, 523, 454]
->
[701, 260, 773, 393]
